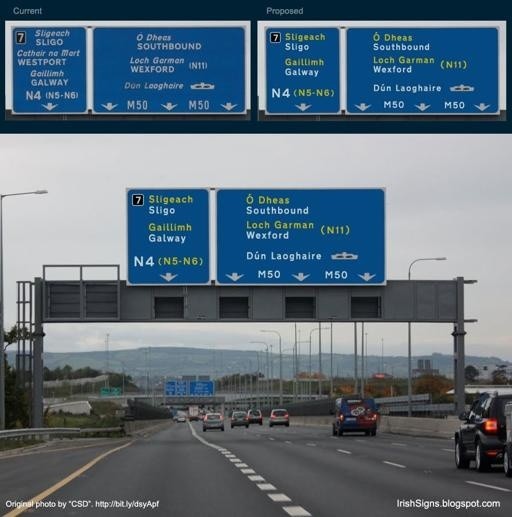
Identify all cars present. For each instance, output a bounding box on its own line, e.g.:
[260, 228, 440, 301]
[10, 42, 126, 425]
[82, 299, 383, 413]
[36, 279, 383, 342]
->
[269, 407, 290, 426]
[173, 415, 177, 420]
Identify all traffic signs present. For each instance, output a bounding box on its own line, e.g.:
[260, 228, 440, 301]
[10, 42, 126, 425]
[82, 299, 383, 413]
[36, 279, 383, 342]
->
[3, 19, 252, 114]
[255, 17, 506, 118]
[189, 380, 214, 396]
[124, 183, 386, 289]
[164, 380, 187, 395]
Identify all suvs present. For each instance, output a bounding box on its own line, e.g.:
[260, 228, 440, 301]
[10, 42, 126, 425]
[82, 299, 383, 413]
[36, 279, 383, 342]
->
[248, 408, 262, 424]
[454, 391, 511, 476]
[202, 412, 224, 431]
[231, 410, 249, 428]
[329, 394, 379, 436]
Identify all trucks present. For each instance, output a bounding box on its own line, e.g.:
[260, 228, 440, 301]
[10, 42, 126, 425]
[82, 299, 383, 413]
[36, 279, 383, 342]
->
[176, 410, 186, 422]
[188, 404, 199, 421]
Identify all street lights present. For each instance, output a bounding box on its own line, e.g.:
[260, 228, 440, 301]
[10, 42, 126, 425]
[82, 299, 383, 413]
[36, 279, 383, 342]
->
[381, 336, 384, 372]
[1, 189, 51, 431]
[365, 332, 368, 383]
[406, 255, 449, 416]
[225, 325, 330, 412]
